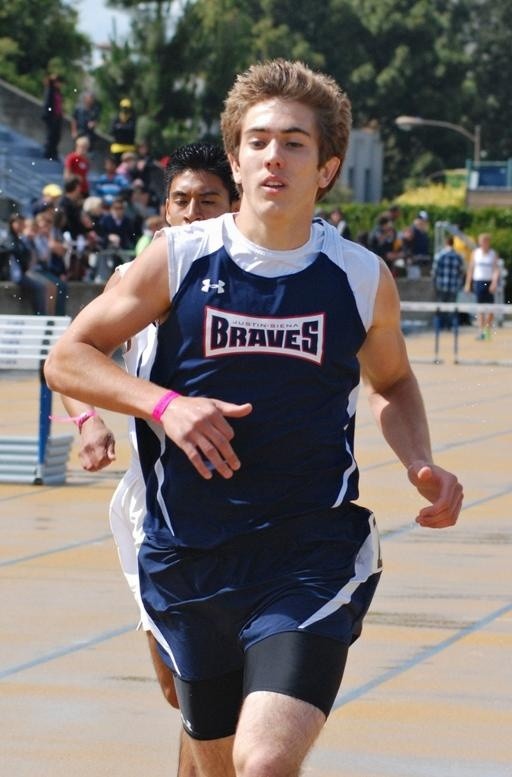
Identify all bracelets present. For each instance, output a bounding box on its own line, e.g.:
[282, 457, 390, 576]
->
[74, 409, 99, 432]
[150, 389, 181, 423]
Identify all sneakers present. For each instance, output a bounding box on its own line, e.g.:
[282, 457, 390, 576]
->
[475, 326, 491, 340]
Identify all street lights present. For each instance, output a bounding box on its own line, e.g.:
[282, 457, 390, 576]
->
[395, 112, 483, 163]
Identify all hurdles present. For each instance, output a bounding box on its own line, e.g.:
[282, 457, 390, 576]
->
[400, 299, 512, 368]
[1, 313, 72, 485]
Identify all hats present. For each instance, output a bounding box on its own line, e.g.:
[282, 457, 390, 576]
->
[32, 203, 54, 215]
[42, 184, 65, 197]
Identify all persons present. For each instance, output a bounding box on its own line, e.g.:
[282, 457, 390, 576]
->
[108, 97, 138, 166]
[426, 235, 468, 331]
[39, 74, 66, 160]
[46, 137, 244, 712]
[1, 136, 169, 285]
[69, 92, 102, 153]
[40, 53, 470, 775]
[314, 204, 433, 283]
[463, 232, 500, 341]
